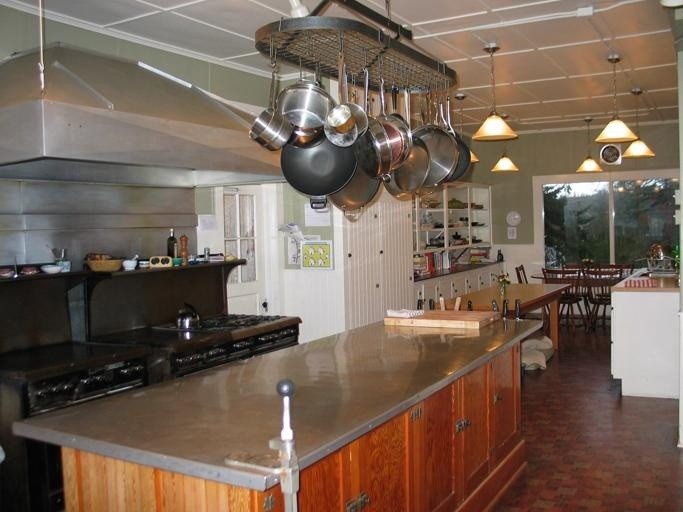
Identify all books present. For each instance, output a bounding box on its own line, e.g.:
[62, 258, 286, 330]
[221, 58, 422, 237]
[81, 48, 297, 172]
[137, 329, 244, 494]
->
[413, 252, 453, 276]
[468, 246, 495, 265]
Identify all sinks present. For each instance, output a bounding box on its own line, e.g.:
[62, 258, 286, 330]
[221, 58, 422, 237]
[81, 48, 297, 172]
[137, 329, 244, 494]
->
[639, 273, 678, 277]
[647, 269, 675, 272]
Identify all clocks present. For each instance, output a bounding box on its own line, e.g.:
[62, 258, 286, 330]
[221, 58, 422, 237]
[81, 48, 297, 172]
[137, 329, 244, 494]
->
[506, 212, 522, 227]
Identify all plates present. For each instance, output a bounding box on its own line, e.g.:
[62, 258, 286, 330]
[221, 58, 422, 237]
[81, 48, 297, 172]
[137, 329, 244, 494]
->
[475, 224, 484, 226]
[473, 240, 482, 243]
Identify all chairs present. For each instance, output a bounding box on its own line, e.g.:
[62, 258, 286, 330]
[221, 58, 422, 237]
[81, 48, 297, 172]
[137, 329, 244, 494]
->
[514, 262, 635, 335]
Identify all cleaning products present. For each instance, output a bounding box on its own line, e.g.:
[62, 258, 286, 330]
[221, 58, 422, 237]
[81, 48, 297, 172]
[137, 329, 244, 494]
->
[671, 245, 679, 271]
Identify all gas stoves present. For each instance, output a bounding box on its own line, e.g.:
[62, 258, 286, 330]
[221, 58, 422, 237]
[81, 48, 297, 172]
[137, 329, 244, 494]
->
[152, 311, 288, 332]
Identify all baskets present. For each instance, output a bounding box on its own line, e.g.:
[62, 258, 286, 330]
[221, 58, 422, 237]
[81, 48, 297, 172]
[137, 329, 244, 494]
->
[82, 256, 125, 273]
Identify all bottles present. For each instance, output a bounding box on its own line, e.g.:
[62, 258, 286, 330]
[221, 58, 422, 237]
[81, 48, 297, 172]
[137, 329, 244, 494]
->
[166, 226, 178, 258]
[204, 248, 210, 261]
[180, 235, 190, 259]
[671, 243, 680, 274]
[497, 250, 503, 263]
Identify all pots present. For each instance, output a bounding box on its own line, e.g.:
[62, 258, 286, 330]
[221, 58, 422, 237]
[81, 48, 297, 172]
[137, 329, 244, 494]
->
[247, 59, 470, 221]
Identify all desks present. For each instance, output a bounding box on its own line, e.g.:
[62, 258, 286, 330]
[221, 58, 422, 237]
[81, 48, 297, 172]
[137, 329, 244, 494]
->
[434, 281, 572, 350]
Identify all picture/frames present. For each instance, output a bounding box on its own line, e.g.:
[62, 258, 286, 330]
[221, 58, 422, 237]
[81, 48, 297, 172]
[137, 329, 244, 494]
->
[300, 239, 333, 270]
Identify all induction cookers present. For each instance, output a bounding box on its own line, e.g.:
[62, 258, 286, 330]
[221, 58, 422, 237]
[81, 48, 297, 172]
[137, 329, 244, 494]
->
[1, 339, 151, 379]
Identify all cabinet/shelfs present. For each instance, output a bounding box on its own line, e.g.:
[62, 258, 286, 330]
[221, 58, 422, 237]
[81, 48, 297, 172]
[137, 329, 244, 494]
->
[414, 180, 507, 312]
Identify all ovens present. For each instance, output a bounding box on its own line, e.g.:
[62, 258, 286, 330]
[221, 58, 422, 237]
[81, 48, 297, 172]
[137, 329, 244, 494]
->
[26, 379, 144, 510]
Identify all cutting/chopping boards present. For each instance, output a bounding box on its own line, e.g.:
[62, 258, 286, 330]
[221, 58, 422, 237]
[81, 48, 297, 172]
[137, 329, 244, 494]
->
[392, 327, 497, 336]
[384, 310, 502, 328]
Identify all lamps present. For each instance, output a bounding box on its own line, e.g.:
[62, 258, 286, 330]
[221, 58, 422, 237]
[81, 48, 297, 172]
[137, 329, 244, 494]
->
[454, 44, 521, 172]
[575, 53, 656, 174]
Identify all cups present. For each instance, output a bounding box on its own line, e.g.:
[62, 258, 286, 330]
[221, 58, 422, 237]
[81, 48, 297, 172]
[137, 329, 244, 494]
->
[57, 259, 71, 273]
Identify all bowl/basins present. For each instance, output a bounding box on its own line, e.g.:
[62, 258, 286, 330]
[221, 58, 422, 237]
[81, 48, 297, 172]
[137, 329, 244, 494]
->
[85, 260, 123, 272]
[463, 202, 484, 209]
[430, 216, 478, 247]
[41, 265, 63, 274]
[139, 261, 150, 268]
[124, 260, 136, 271]
[172, 258, 182, 268]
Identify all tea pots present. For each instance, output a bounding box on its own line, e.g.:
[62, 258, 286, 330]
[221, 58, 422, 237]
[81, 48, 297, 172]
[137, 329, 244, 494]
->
[175, 303, 201, 329]
